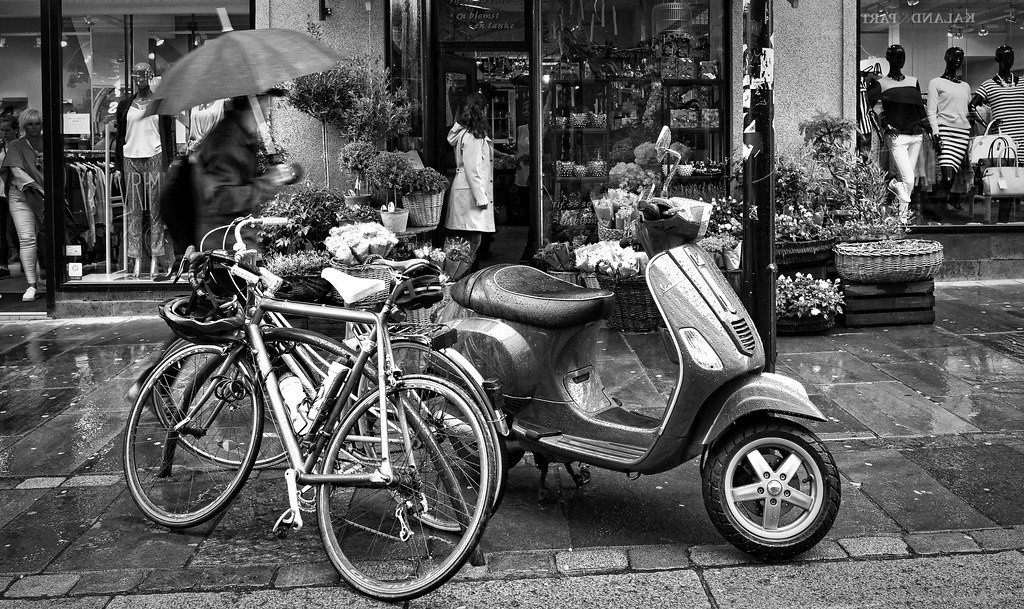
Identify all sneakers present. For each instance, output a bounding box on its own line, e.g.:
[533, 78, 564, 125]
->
[38, 277, 47, 289]
[22, 287, 40, 301]
[125, 379, 182, 426]
[0, 267, 11, 280]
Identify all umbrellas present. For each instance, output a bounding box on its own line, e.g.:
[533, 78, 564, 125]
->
[136, 7, 348, 185]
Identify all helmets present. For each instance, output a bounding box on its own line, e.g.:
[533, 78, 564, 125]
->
[158, 296, 247, 345]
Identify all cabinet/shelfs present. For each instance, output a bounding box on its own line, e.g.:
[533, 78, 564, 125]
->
[446, 51, 725, 251]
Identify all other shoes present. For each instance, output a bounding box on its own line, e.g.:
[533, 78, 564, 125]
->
[519, 245, 539, 263]
[489, 241, 496, 254]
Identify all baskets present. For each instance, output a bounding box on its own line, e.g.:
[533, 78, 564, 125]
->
[329, 253, 391, 306]
[773, 231, 947, 280]
[545, 225, 664, 332]
[401, 187, 445, 226]
[404, 284, 460, 324]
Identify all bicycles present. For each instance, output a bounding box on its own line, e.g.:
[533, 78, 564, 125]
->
[120, 213, 513, 602]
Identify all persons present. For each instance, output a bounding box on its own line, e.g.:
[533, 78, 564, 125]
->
[446, 93, 530, 256]
[185, 96, 230, 157]
[0, 109, 46, 301]
[967, 45, 1024, 166]
[928, 47, 972, 223]
[867, 44, 935, 221]
[113, 64, 170, 278]
[124, 86, 293, 419]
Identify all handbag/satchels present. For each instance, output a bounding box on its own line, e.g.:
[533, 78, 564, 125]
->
[970, 119, 1018, 163]
[982, 147, 1024, 196]
[976, 137, 1019, 186]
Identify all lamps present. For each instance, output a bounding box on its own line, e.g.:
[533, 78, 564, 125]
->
[861, 0, 1024, 40]
[0, 30, 207, 90]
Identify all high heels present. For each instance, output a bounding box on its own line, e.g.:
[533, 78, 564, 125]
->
[154, 260, 180, 282]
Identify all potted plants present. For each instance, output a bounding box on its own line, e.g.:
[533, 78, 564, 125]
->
[795, 107, 936, 326]
[259, 57, 476, 286]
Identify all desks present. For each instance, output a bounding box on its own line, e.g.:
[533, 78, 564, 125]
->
[397, 225, 440, 240]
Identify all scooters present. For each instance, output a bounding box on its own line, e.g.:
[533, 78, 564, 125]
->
[411, 124, 845, 563]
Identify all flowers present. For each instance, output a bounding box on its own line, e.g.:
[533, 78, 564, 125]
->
[535, 143, 842, 322]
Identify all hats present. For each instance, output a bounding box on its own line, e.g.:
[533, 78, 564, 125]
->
[233, 87, 288, 110]
